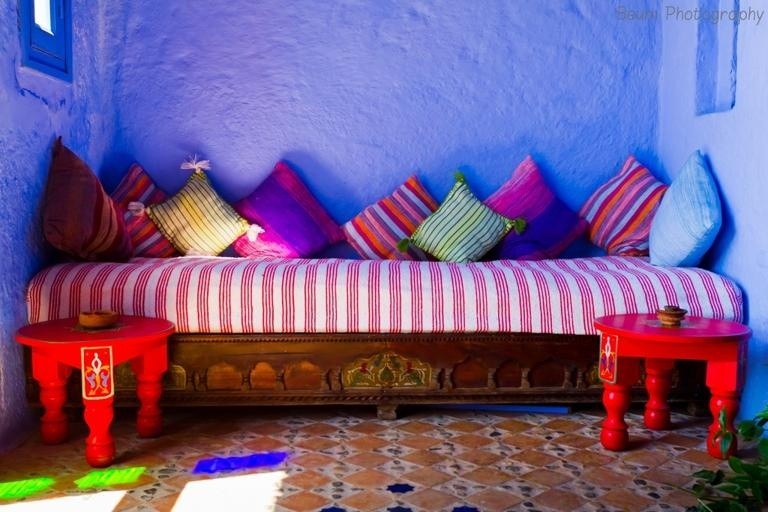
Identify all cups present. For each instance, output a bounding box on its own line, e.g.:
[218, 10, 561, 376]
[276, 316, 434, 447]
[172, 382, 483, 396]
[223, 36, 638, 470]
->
[656, 305, 688, 327]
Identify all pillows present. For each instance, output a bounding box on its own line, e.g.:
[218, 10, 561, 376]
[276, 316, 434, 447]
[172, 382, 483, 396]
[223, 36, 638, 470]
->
[230, 160, 346, 259]
[43, 136, 136, 262]
[482, 156, 589, 261]
[397, 169, 526, 264]
[649, 150, 722, 268]
[127, 154, 265, 257]
[110, 161, 177, 257]
[339, 172, 440, 260]
[577, 156, 668, 255]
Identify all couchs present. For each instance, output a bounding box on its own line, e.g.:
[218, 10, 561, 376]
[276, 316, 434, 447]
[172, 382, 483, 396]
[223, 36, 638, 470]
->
[27, 257, 742, 420]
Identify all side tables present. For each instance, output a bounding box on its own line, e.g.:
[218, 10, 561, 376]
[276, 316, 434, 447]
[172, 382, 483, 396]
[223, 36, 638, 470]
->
[594, 313, 753, 461]
[13, 316, 176, 468]
